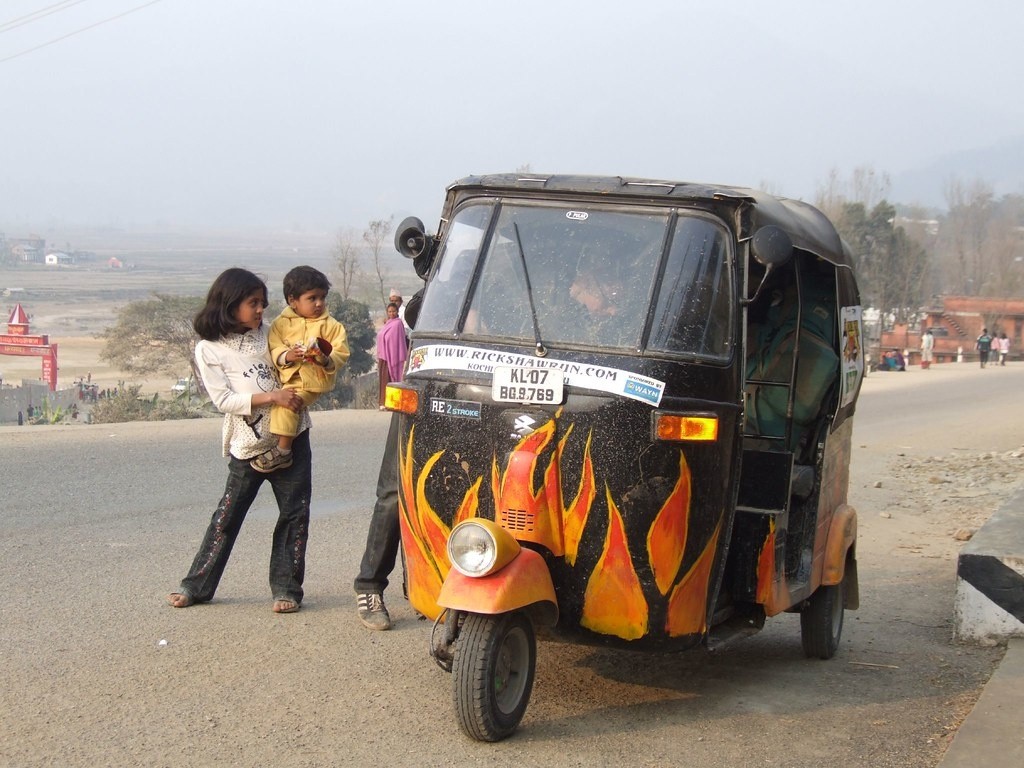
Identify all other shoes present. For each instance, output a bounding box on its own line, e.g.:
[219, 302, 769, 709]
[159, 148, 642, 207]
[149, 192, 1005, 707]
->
[379, 405, 386, 411]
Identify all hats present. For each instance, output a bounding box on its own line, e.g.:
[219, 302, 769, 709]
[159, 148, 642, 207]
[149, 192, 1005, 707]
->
[389, 289, 403, 299]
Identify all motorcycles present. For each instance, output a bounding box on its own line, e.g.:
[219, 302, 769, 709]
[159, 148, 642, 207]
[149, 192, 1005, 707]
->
[394, 171, 868, 741]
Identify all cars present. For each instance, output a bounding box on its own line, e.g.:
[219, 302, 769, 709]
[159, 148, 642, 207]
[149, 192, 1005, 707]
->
[171, 378, 197, 397]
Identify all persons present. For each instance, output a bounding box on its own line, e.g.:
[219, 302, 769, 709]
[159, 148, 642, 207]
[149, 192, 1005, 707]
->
[78, 373, 125, 402]
[250, 266, 350, 473]
[460, 258, 632, 334]
[383, 288, 412, 347]
[73, 404, 79, 418]
[353, 244, 517, 631]
[975, 328, 992, 368]
[990, 333, 1010, 366]
[920, 330, 934, 368]
[166, 268, 312, 613]
[883, 347, 908, 371]
[18, 411, 23, 426]
[27, 404, 41, 420]
[379, 303, 408, 411]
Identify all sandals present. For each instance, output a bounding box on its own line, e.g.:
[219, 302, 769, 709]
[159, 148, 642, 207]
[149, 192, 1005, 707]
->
[249, 445, 294, 473]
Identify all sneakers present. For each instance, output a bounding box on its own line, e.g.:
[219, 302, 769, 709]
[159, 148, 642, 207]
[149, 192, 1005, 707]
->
[356, 592, 390, 630]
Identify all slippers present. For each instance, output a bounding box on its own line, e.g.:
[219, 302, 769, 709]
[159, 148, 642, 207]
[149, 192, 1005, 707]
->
[273, 594, 298, 613]
[167, 586, 196, 607]
